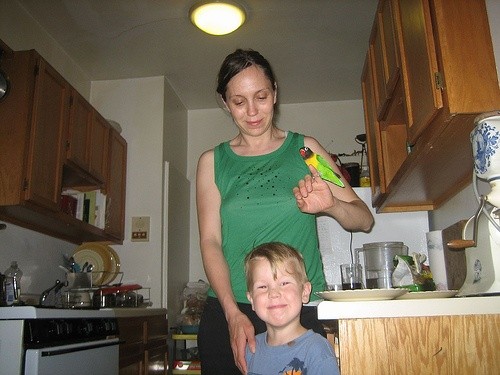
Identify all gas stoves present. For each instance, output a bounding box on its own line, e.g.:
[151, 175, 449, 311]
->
[0, 300, 116, 320]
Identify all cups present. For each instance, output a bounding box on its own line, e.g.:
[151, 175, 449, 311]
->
[340, 263, 363, 291]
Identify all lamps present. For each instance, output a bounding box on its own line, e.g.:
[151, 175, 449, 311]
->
[186, 0, 251, 38]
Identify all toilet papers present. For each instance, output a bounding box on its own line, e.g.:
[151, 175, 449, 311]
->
[426, 230, 448, 291]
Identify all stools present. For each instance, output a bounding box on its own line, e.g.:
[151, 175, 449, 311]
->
[172, 333, 198, 366]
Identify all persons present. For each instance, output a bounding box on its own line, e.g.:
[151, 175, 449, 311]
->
[196, 48, 375, 375]
[243, 241, 341, 375]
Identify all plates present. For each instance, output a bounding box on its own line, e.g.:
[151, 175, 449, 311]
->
[395, 290, 460, 299]
[71, 243, 120, 285]
[313, 288, 409, 301]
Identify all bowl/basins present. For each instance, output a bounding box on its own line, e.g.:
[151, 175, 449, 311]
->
[180, 326, 199, 334]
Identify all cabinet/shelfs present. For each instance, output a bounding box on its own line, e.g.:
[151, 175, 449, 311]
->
[361, 0, 500, 214]
[0, 49, 128, 246]
[119, 315, 168, 375]
[317, 296, 500, 375]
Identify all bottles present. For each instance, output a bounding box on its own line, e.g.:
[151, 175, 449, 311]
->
[4, 261, 22, 305]
[359, 166, 370, 187]
[93, 284, 135, 308]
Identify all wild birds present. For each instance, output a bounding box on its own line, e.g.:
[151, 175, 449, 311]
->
[300, 146, 346, 189]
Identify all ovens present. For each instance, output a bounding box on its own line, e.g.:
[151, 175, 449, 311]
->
[0, 319, 120, 375]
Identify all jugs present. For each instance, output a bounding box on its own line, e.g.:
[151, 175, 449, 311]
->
[355, 242, 408, 289]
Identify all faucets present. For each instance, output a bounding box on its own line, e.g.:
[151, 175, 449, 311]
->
[37, 280, 69, 305]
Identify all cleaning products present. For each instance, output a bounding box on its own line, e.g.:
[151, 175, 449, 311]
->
[3, 260, 24, 306]
